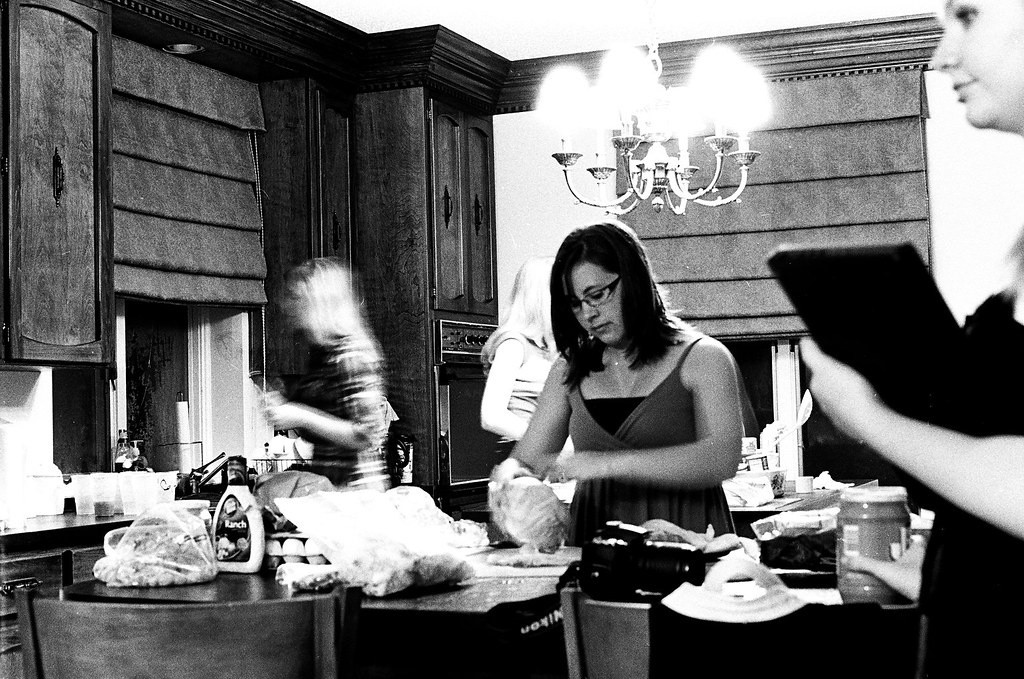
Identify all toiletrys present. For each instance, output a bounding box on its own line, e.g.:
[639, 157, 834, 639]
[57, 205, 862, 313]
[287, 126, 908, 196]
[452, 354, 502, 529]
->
[209, 456, 265, 573]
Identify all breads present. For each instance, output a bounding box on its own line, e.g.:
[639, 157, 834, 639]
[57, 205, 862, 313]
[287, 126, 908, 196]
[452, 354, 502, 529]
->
[499, 476, 569, 552]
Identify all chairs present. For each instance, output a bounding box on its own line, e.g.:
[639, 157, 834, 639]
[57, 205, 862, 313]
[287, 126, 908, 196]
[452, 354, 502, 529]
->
[16, 579, 929, 679]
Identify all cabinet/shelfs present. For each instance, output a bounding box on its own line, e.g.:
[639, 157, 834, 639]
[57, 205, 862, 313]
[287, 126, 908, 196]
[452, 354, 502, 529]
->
[2, 1, 534, 365]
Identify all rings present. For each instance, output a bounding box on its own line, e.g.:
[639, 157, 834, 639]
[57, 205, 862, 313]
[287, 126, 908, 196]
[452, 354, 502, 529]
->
[558, 472, 568, 483]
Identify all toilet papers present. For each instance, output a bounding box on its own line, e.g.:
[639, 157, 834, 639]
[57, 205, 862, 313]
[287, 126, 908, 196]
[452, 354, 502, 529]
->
[172, 401, 193, 475]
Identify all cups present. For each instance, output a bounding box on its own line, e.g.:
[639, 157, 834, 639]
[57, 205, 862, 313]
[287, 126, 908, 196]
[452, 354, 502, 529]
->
[63, 498, 76, 513]
[745, 453, 779, 470]
[741, 437, 758, 456]
[796, 477, 813, 493]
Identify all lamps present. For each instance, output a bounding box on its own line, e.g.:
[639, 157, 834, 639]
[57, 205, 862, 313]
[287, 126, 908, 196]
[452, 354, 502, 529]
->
[536, 1, 772, 211]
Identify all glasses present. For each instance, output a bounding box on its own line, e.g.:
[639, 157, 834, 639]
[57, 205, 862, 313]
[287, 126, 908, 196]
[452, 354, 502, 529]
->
[569, 275, 622, 314]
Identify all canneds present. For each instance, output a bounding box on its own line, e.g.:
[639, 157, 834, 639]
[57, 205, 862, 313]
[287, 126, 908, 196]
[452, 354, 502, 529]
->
[834, 485, 911, 604]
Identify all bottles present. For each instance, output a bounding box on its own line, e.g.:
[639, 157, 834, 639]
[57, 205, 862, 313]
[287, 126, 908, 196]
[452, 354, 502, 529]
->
[211, 477, 265, 573]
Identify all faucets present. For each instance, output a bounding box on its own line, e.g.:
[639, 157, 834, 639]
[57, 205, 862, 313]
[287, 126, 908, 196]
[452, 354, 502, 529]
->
[188, 453, 229, 493]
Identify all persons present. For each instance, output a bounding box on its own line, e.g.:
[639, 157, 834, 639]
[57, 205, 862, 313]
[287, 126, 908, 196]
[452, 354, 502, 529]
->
[799, 1, 1024, 678]
[260, 258, 392, 492]
[481, 220, 742, 538]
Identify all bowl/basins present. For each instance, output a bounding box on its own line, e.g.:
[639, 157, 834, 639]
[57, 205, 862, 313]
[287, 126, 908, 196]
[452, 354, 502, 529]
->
[736, 469, 785, 495]
